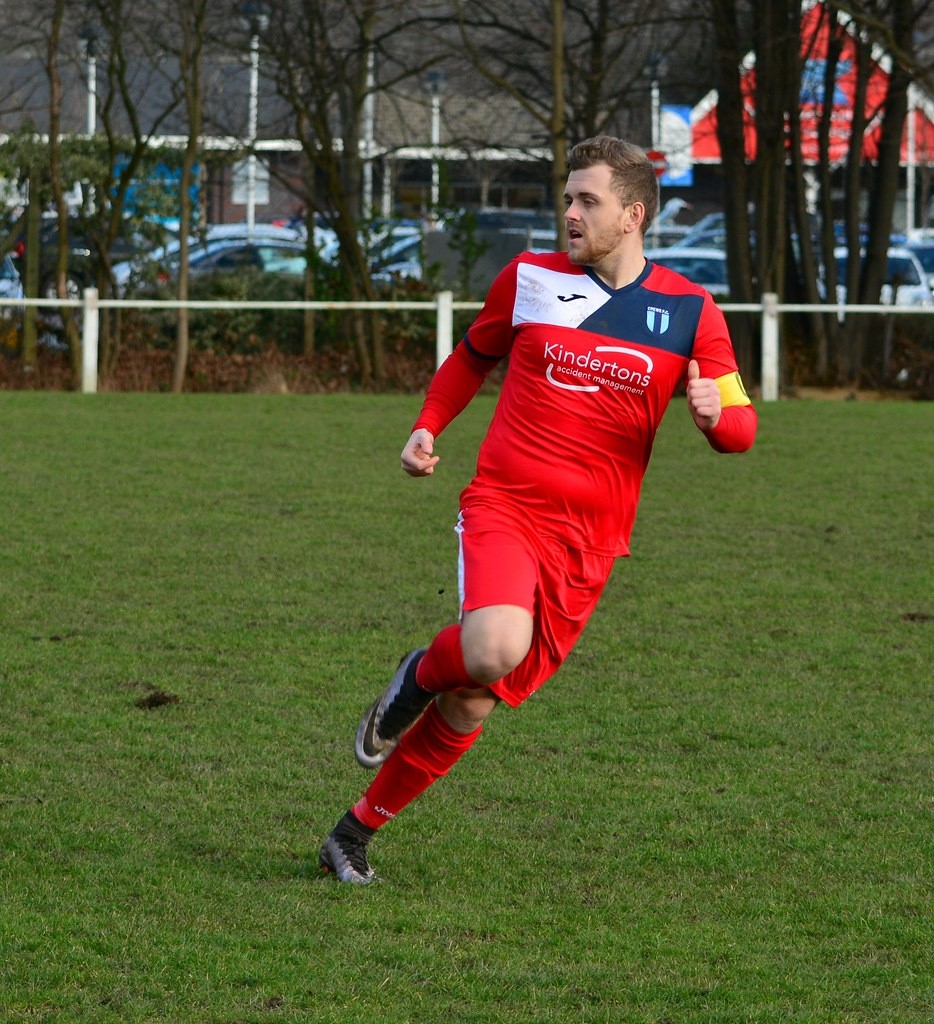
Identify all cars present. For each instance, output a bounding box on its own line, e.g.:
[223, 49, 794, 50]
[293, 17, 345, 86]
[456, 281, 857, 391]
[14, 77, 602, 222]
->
[0, 211, 932, 345]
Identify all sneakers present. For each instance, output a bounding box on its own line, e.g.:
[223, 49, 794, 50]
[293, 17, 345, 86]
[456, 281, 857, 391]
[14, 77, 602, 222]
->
[318, 829, 374, 887]
[353, 648, 436, 768]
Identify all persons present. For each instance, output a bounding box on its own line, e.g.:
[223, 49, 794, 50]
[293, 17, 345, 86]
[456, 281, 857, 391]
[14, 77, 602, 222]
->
[316, 135, 764, 881]
